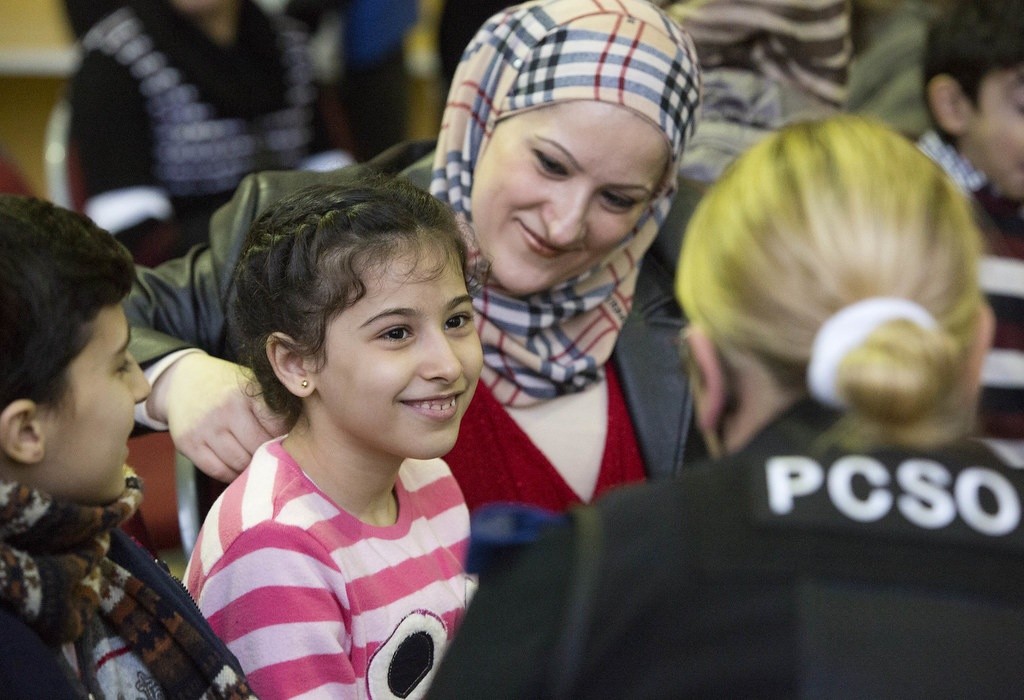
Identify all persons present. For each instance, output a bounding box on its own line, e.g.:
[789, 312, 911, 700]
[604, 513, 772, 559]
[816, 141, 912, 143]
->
[0, 189, 263, 700]
[175, 169, 482, 700]
[282, 1, 534, 174]
[419, 116, 1024, 699]
[653, 0, 933, 142]
[59, 0, 360, 271]
[120, 0, 723, 585]
[895, 0, 1024, 476]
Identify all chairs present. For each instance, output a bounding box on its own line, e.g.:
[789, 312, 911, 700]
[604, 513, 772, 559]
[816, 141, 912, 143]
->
[46, 77, 363, 270]
